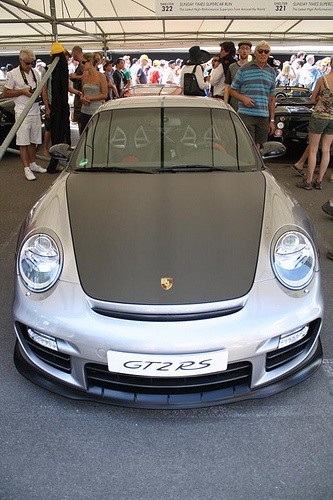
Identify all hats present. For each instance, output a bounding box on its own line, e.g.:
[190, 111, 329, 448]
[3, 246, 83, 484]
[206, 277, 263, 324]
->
[238, 40, 252, 47]
[51, 42, 64, 54]
[140, 55, 148, 61]
[102, 59, 113, 71]
[183, 46, 212, 65]
[153, 60, 160, 66]
[169, 60, 176, 64]
[176, 59, 183, 64]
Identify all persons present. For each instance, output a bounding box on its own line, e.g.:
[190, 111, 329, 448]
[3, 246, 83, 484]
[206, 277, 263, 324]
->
[70, 46, 82, 122]
[210, 42, 237, 100]
[227, 40, 276, 150]
[0, 54, 183, 124]
[321, 198, 333, 259]
[203, 55, 252, 95]
[42, 49, 82, 157]
[3, 49, 50, 181]
[267, 52, 332, 92]
[291, 58, 333, 190]
[181, 46, 209, 96]
[224, 41, 252, 112]
[80, 54, 107, 135]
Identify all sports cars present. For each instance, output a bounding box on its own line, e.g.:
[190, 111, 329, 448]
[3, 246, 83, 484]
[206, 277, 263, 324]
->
[10, 94, 325, 411]
[268, 86, 314, 148]
[118, 83, 184, 95]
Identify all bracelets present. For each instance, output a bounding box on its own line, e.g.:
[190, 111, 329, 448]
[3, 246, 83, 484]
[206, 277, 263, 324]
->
[270, 120, 274, 122]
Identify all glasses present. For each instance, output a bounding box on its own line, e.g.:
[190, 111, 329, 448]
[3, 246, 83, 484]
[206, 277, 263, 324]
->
[211, 59, 219, 63]
[256, 49, 269, 54]
[82, 60, 90, 65]
[23, 60, 34, 66]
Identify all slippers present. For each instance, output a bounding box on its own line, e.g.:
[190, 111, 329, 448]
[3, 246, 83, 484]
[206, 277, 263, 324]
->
[314, 170, 326, 174]
[291, 164, 304, 176]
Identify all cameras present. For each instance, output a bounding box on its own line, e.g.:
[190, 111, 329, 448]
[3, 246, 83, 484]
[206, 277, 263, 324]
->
[30, 88, 39, 100]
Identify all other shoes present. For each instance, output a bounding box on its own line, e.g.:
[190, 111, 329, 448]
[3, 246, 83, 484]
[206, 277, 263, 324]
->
[312, 181, 322, 190]
[321, 201, 333, 216]
[30, 165, 47, 173]
[296, 182, 312, 191]
[25, 170, 36, 180]
